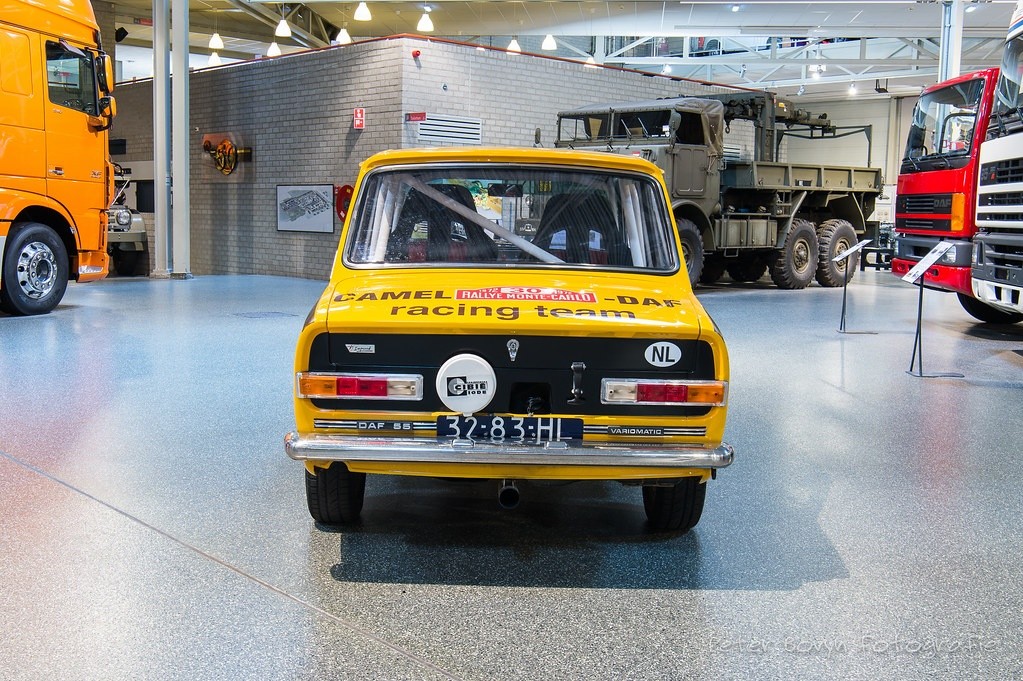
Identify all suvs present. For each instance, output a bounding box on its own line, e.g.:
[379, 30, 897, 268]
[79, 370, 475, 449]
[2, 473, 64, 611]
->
[106, 178, 148, 275]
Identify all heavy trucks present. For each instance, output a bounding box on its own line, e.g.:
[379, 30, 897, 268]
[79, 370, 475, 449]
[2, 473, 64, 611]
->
[971, 1, 1023, 313]
[0, 0, 123, 316]
[532, 89, 884, 291]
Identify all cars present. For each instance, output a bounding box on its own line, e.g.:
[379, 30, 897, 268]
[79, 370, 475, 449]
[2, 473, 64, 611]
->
[285, 145, 733, 536]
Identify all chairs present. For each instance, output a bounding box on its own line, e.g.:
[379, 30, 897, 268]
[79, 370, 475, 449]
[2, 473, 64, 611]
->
[387, 184, 498, 261]
[528, 193, 623, 264]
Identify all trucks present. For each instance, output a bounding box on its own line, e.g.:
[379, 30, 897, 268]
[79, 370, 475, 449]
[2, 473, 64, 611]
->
[891, 66, 1022, 327]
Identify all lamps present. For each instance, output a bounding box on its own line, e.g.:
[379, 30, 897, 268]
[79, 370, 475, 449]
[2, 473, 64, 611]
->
[417, 0, 434, 32]
[739, 65, 746, 78]
[209, 13, 224, 50]
[336, 3, 352, 44]
[267, 30, 282, 57]
[541, 34, 557, 50]
[815, 42, 822, 59]
[209, 50, 221, 66]
[506, 35, 522, 55]
[275, 3, 291, 38]
[797, 86, 804, 96]
[354, 1, 372, 22]
